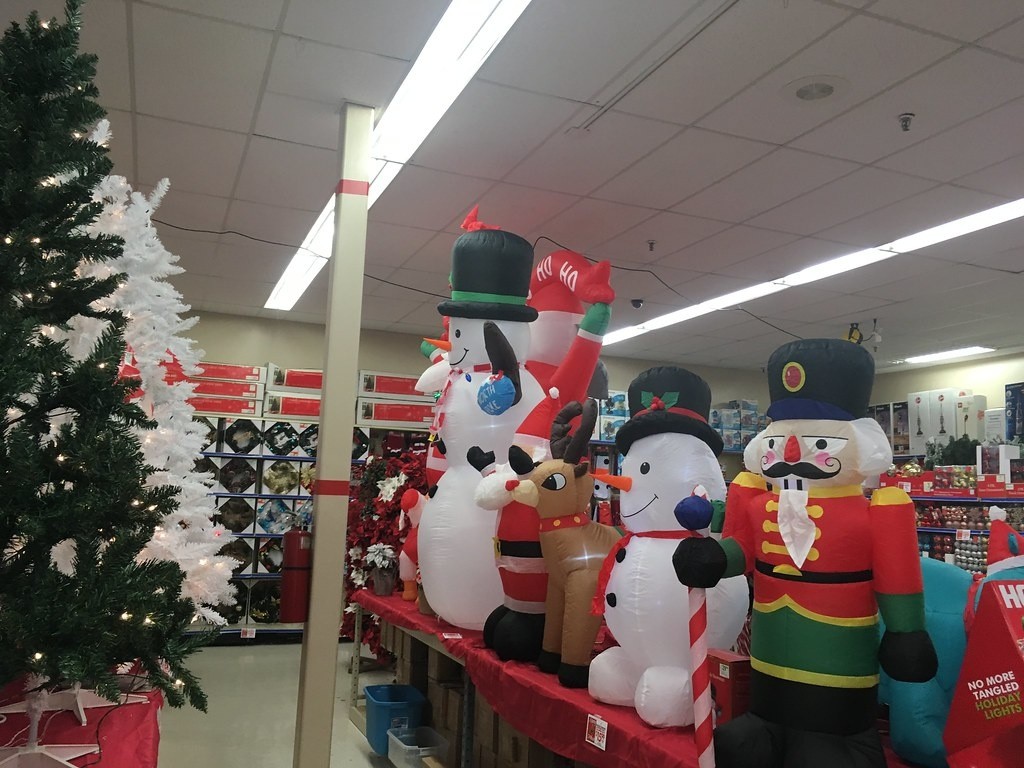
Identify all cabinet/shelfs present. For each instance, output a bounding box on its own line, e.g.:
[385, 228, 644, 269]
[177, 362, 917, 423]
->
[911, 497, 1024, 537]
[178, 411, 432, 641]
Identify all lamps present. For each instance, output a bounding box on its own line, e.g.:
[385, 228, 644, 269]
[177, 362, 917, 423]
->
[264, 0, 531, 311]
[599, 197, 1024, 346]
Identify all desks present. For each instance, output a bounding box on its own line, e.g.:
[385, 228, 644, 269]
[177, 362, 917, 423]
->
[0, 660, 163, 768]
[350, 587, 917, 767]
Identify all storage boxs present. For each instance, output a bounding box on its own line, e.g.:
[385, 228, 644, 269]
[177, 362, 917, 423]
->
[589, 390, 633, 500]
[865, 383, 1024, 500]
[707, 647, 750, 728]
[121, 339, 439, 625]
[708, 399, 771, 451]
[380, 615, 548, 768]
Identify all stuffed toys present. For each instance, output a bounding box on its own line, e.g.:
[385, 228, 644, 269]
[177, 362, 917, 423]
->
[588, 367, 750, 727]
[396, 205, 615, 660]
[673, 339, 938, 768]
[506, 399, 629, 688]
[878, 507, 1024, 768]
